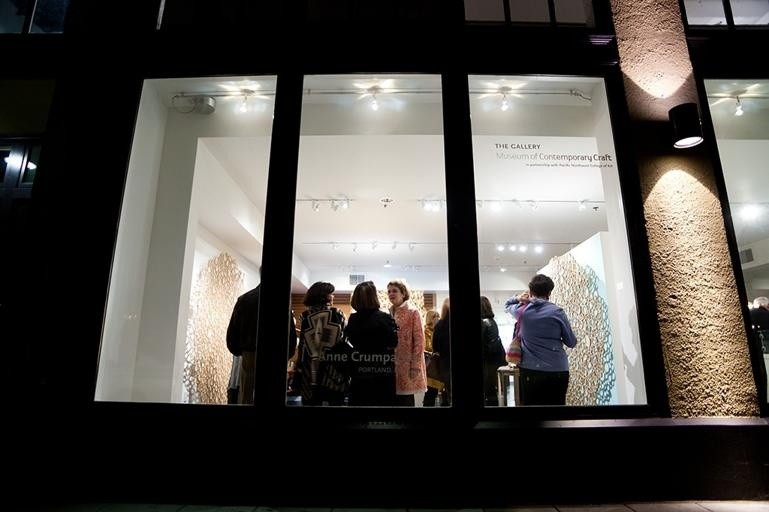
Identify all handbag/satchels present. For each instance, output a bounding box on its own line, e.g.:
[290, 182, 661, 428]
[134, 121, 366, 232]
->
[325, 338, 358, 380]
[504, 336, 523, 365]
[423, 350, 445, 383]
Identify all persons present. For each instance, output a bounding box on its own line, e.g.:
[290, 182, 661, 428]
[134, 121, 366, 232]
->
[386, 281, 429, 405]
[503, 274, 577, 405]
[749, 296, 769, 330]
[480, 295, 506, 406]
[431, 297, 450, 393]
[225, 266, 298, 404]
[287, 281, 348, 406]
[348, 280, 398, 406]
[423, 308, 441, 406]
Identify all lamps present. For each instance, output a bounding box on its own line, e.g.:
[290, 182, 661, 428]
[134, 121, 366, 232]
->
[669, 102, 705, 151]
[708, 92, 769, 117]
[171, 88, 579, 112]
[297, 198, 356, 211]
[302, 242, 417, 252]
[383, 260, 392, 267]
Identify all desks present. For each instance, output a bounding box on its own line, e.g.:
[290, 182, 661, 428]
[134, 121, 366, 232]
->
[497, 366, 521, 406]
